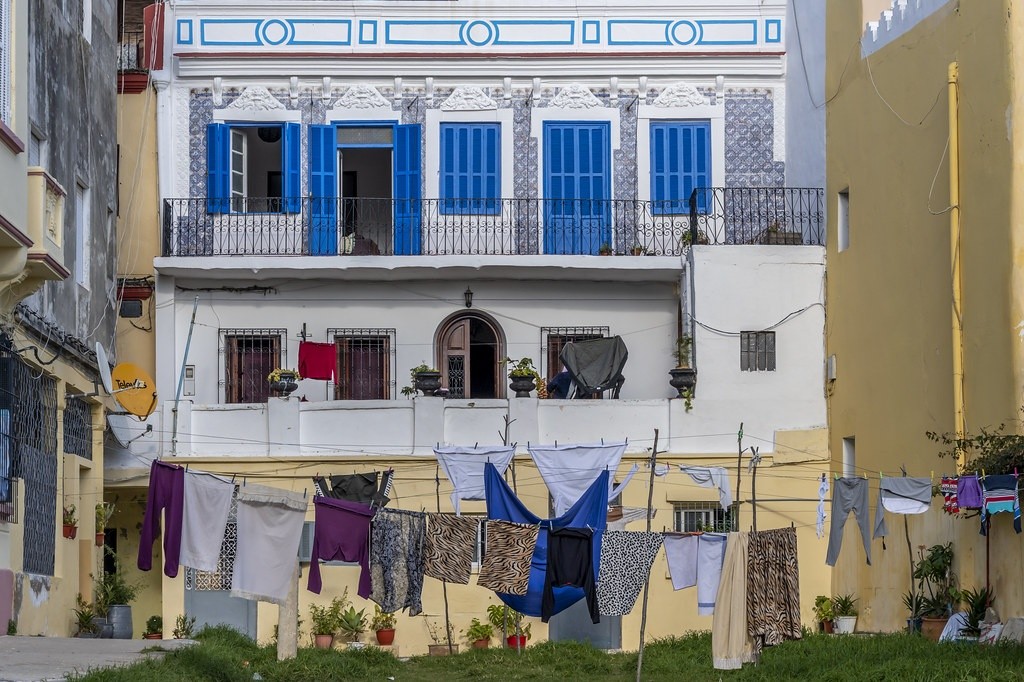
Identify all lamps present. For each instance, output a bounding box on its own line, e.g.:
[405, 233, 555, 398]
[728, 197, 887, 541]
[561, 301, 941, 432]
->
[464, 285, 474, 308]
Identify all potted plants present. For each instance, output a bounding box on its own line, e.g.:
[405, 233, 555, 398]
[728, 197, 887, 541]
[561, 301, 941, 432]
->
[338, 605, 368, 652]
[267, 368, 301, 397]
[459, 618, 494, 649]
[71, 572, 141, 639]
[308, 586, 353, 650]
[63, 504, 78, 539]
[501, 356, 542, 397]
[812, 592, 861, 634]
[410, 364, 442, 397]
[95, 502, 115, 546]
[600, 229, 707, 256]
[369, 604, 397, 645]
[487, 604, 531, 649]
[903, 542, 997, 643]
[667, 333, 697, 412]
[141, 615, 162, 639]
[422, 611, 459, 656]
